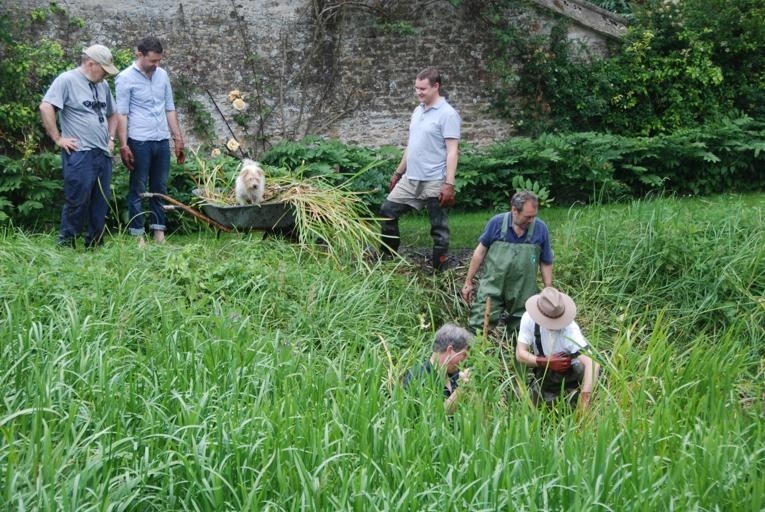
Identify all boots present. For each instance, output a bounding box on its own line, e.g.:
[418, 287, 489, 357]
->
[433, 245, 448, 272]
[372, 231, 401, 259]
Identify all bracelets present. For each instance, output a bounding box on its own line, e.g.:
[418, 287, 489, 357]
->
[109, 136, 115, 141]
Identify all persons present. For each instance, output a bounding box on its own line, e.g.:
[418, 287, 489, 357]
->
[368, 66, 463, 276]
[513, 285, 600, 417]
[114, 34, 186, 247]
[400, 323, 474, 437]
[461, 190, 554, 404]
[40, 43, 117, 249]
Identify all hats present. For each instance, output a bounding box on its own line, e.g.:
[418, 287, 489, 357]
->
[81, 45, 120, 75]
[526, 287, 577, 330]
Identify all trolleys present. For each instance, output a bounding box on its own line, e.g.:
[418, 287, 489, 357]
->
[139, 189, 332, 244]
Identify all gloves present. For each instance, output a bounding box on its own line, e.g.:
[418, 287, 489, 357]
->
[175, 140, 185, 164]
[120, 146, 134, 170]
[391, 171, 401, 190]
[578, 392, 591, 415]
[439, 183, 455, 208]
[537, 352, 571, 372]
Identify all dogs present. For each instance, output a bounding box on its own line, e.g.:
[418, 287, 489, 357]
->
[235, 158, 266, 206]
